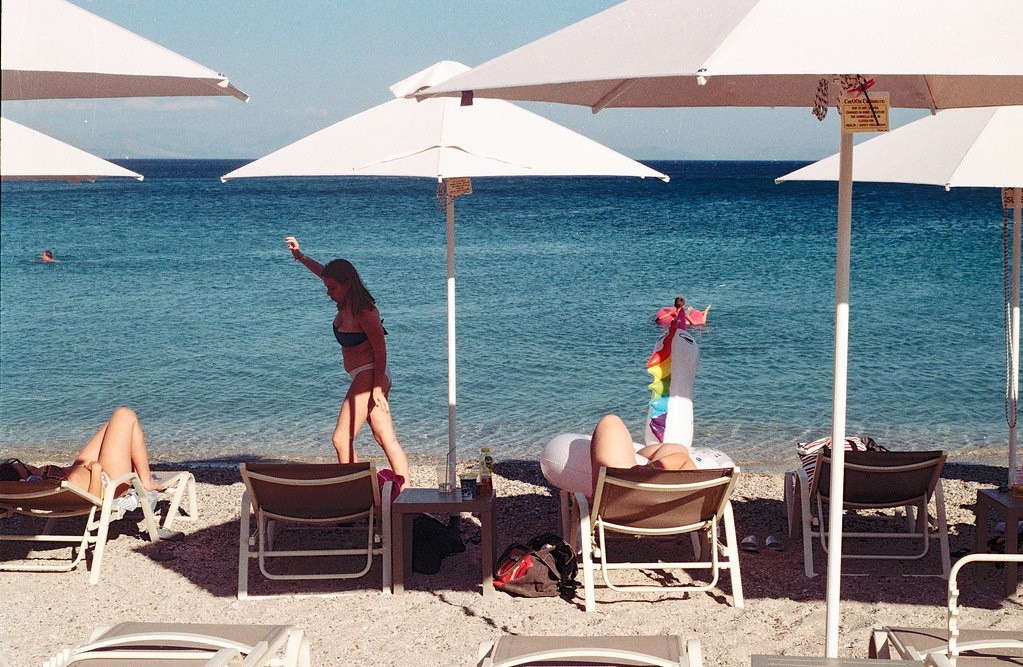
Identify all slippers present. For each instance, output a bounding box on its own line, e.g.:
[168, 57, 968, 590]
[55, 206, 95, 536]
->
[158, 526, 185, 541]
[766, 535, 784, 551]
[150, 547, 176, 561]
[740, 536, 760, 554]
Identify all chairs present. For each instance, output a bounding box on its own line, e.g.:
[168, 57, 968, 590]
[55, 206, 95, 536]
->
[477, 635, 702, 667]
[44, 622, 309, 667]
[0, 469, 195, 581]
[784, 445, 952, 582]
[869, 626, 1023, 667]
[238, 463, 399, 596]
[557, 465, 743, 612]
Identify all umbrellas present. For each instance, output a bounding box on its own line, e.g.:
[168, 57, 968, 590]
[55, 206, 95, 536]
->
[1, 116, 144, 182]
[400, 0, 1023, 657]
[220, 56, 669, 491]
[1, 0, 254, 103]
[774, 103, 1022, 487]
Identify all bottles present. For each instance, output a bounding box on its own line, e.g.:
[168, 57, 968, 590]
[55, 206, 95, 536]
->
[1012, 469, 1023, 497]
[478, 447, 493, 497]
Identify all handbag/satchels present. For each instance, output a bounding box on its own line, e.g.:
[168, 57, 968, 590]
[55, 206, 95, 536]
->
[413, 514, 465, 575]
[493, 532, 578, 598]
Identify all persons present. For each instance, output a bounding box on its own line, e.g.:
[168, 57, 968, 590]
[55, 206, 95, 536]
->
[285, 237, 410, 492]
[591, 413, 696, 497]
[0, 408, 166, 509]
[42, 251, 55, 262]
[657, 297, 686, 321]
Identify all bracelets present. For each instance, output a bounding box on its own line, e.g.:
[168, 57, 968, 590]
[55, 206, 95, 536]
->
[295, 253, 306, 262]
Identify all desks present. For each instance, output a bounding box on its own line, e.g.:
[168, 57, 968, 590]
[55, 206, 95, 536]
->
[392, 489, 494, 597]
[750, 652, 922, 667]
[977, 487, 1023, 601]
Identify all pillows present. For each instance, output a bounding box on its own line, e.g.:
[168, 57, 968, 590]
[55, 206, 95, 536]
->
[798, 434, 870, 488]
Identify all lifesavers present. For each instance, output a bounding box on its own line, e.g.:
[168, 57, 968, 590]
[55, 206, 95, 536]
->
[655, 306, 710, 328]
[539, 320, 738, 498]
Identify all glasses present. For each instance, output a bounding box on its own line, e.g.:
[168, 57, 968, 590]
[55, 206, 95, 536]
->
[11, 459, 33, 478]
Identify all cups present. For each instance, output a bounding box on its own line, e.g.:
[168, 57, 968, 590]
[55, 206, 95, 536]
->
[457, 473, 478, 501]
[436, 460, 455, 493]
